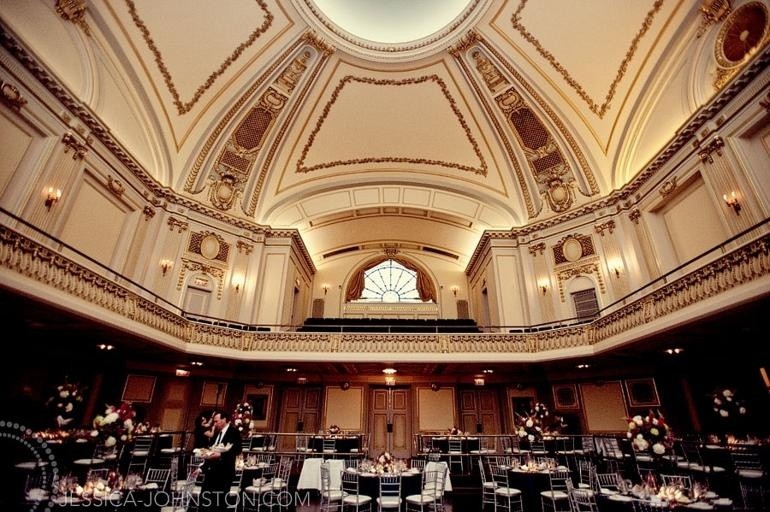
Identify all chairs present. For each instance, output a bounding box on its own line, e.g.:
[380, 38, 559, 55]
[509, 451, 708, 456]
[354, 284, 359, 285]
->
[2, 411, 769, 510]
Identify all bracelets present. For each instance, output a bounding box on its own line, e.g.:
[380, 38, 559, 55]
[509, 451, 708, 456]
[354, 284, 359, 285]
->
[210, 417, 213, 418]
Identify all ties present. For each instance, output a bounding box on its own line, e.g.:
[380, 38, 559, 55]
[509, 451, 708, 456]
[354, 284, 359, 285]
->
[217, 432, 222, 445]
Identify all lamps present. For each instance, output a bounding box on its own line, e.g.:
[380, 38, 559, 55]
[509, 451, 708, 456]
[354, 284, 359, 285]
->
[322, 282, 328, 295]
[723, 190, 743, 216]
[450, 284, 461, 299]
[159, 258, 172, 278]
[609, 256, 625, 277]
[539, 279, 549, 296]
[41, 186, 64, 209]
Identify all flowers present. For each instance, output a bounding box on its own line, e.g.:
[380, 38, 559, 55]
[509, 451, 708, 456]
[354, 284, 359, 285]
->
[709, 388, 749, 418]
[91, 399, 151, 455]
[233, 400, 257, 438]
[532, 400, 549, 421]
[47, 381, 85, 421]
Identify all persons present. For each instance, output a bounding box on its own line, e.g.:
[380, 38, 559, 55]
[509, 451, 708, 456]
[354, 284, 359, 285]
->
[194, 413, 244, 512]
[195, 411, 216, 446]
